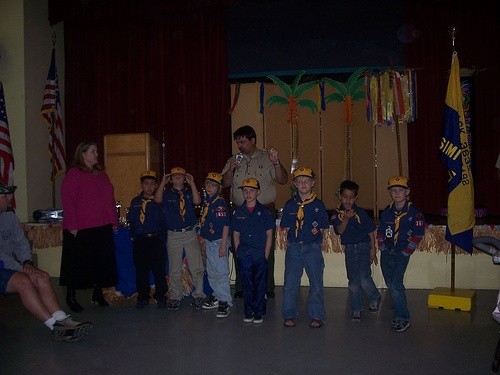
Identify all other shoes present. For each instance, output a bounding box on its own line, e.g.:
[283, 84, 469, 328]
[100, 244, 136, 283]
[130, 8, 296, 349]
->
[310, 318, 322, 328]
[284, 318, 296, 327]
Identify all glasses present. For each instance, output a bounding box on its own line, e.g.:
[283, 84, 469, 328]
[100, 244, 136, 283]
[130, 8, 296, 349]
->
[298, 180, 310, 183]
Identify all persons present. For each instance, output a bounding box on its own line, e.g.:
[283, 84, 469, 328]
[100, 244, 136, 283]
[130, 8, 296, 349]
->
[129, 170, 169, 309]
[279, 167, 330, 328]
[154, 167, 206, 312]
[376, 176, 424, 332]
[332, 180, 381, 323]
[59, 140, 118, 313]
[0, 182, 96, 342]
[220, 125, 288, 302]
[231, 178, 277, 322]
[200, 172, 232, 318]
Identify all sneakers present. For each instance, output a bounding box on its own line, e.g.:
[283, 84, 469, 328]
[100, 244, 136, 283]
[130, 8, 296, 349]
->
[351, 310, 361, 322]
[51, 314, 95, 342]
[368, 296, 381, 313]
[392, 320, 410, 332]
[138, 290, 275, 323]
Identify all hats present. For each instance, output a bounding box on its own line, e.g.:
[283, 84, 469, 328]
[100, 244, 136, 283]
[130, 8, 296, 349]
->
[168, 167, 186, 176]
[387, 176, 408, 190]
[238, 178, 260, 190]
[291, 167, 314, 181]
[206, 172, 224, 185]
[140, 171, 156, 179]
[0, 183, 17, 194]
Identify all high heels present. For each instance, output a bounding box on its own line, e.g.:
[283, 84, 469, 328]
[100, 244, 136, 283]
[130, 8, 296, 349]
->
[92, 289, 110, 307]
[66, 291, 83, 313]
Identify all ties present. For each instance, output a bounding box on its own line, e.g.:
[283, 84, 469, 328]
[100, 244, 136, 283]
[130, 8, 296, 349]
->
[139, 199, 154, 224]
[200, 194, 222, 227]
[293, 194, 317, 237]
[179, 190, 185, 222]
[389, 199, 412, 247]
[336, 204, 361, 225]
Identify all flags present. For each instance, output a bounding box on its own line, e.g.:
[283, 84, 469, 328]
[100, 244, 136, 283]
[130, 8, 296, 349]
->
[0, 80, 16, 213]
[41, 46, 68, 182]
[439, 49, 476, 255]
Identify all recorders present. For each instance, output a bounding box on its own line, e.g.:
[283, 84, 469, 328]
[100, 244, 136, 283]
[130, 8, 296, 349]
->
[32, 208, 64, 223]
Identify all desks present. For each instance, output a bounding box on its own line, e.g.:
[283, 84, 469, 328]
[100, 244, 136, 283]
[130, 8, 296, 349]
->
[275, 220, 500, 292]
[22, 222, 64, 278]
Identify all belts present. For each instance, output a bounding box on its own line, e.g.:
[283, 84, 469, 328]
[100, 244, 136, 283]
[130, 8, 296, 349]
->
[168, 225, 194, 232]
[135, 233, 158, 238]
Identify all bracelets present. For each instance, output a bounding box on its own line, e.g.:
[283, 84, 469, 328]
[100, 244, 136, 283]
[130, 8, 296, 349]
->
[273, 161, 280, 165]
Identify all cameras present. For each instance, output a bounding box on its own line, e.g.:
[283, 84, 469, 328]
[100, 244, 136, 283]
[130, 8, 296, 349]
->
[236, 152, 244, 165]
